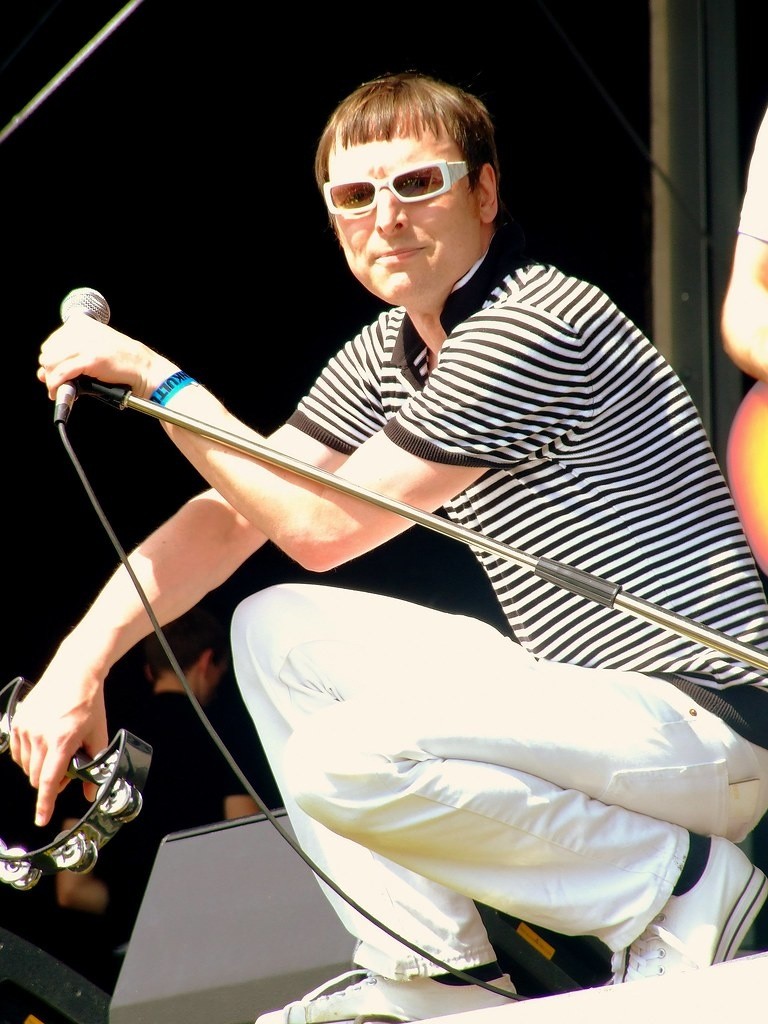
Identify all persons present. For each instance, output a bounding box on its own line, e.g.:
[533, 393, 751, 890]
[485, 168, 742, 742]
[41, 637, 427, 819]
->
[10, 77, 768, 1024]
[720, 107, 768, 380]
[54, 607, 269, 920]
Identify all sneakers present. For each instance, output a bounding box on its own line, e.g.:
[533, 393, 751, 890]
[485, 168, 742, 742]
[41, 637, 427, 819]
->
[613, 832, 768, 986]
[253, 967, 515, 1024]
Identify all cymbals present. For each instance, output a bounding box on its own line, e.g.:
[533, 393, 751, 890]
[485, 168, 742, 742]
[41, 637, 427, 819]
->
[0, 675, 155, 891]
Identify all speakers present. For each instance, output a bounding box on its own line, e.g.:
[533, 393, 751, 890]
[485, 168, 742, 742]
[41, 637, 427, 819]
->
[103, 809, 367, 1024]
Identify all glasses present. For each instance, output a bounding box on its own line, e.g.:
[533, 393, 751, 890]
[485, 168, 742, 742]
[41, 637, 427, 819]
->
[318, 157, 472, 218]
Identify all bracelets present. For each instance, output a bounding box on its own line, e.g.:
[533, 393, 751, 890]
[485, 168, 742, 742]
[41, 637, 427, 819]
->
[150, 371, 195, 406]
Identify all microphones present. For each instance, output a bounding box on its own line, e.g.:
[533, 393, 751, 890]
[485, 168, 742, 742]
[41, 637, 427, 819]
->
[53, 287, 111, 428]
